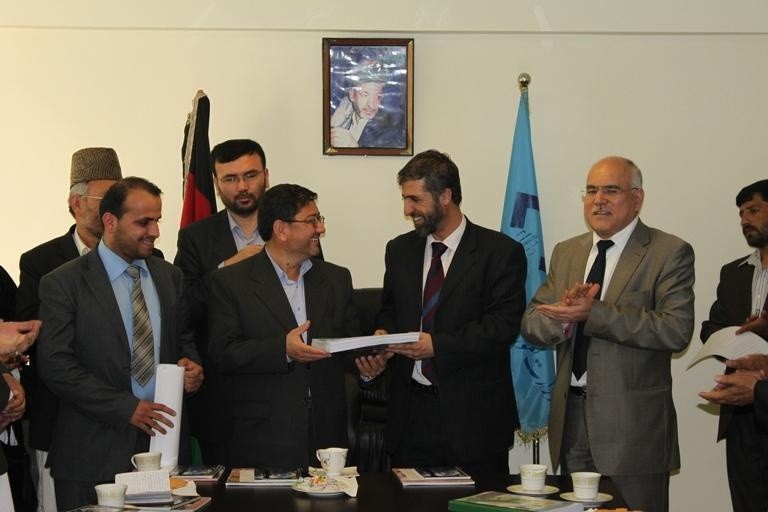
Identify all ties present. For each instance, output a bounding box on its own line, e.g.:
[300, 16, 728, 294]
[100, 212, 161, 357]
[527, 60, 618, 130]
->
[123, 264, 155, 389]
[420, 240, 450, 389]
[571, 239, 617, 382]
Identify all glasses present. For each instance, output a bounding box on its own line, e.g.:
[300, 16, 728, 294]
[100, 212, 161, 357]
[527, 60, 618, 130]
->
[217, 168, 265, 184]
[288, 214, 326, 225]
[579, 186, 639, 204]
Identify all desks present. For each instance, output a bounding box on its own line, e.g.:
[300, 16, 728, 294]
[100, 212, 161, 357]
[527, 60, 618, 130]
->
[83, 468, 633, 511]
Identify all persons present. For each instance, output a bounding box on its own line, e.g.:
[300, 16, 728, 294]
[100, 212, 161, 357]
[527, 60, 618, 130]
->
[1, 317, 43, 358]
[373, 150, 528, 468]
[173, 139, 326, 451]
[699, 351, 768, 417]
[330, 63, 408, 149]
[17, 146, 166, 509]
[518, 157, 693, 512]
[1, 262, 44, 511]
[33, 177, 207, 510]
[699, 176, 767, 511]
[205, 183, 389, 473]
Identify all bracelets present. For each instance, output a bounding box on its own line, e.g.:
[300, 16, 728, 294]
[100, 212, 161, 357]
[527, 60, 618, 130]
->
[361, 373, 376, 379]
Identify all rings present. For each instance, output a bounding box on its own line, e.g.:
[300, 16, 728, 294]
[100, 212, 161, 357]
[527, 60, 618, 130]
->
[14, 350, 21, 360]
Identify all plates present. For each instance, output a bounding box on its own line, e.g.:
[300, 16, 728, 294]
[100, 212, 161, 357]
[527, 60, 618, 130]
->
[309, 465, 360, 478]
[560, 491, 614, 507]
[292, 486, 343, 496]
[506, 484, 559, 498]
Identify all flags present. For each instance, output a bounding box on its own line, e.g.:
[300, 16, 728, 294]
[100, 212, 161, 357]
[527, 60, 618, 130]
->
[500, 92, 557, 441]
[179, 91, 218, 230]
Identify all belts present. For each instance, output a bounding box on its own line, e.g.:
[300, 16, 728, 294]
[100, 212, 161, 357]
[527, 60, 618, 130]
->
[305, 397, 313, 410]
[567, 385, 589, 402]
[410, 377, 440, 397]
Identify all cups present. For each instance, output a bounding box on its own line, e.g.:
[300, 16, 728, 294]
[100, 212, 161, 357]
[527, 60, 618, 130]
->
[316, 447, 348, 476]
[95, 484, 128, 508]
[130, 451, 162, 471]
[520, 463, 547, 491]
[570, 472, 602, 500]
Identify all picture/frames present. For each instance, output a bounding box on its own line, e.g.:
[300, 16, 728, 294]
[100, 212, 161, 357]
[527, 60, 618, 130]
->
[322, 36, 416, 158]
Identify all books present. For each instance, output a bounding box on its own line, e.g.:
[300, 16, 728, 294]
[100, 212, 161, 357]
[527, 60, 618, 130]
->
[310, 332, 421, 355]
[165, 461, 225, 482]
[224, 465, 302, 486]
[448, 489, 584, 512]
[393, 462, 476, 488]
[72, 493, 213, 511]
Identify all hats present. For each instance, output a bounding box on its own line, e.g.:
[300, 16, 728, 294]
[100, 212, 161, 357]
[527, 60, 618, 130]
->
[70, 146, 124, 191]
[343, 62, 389, 89]
